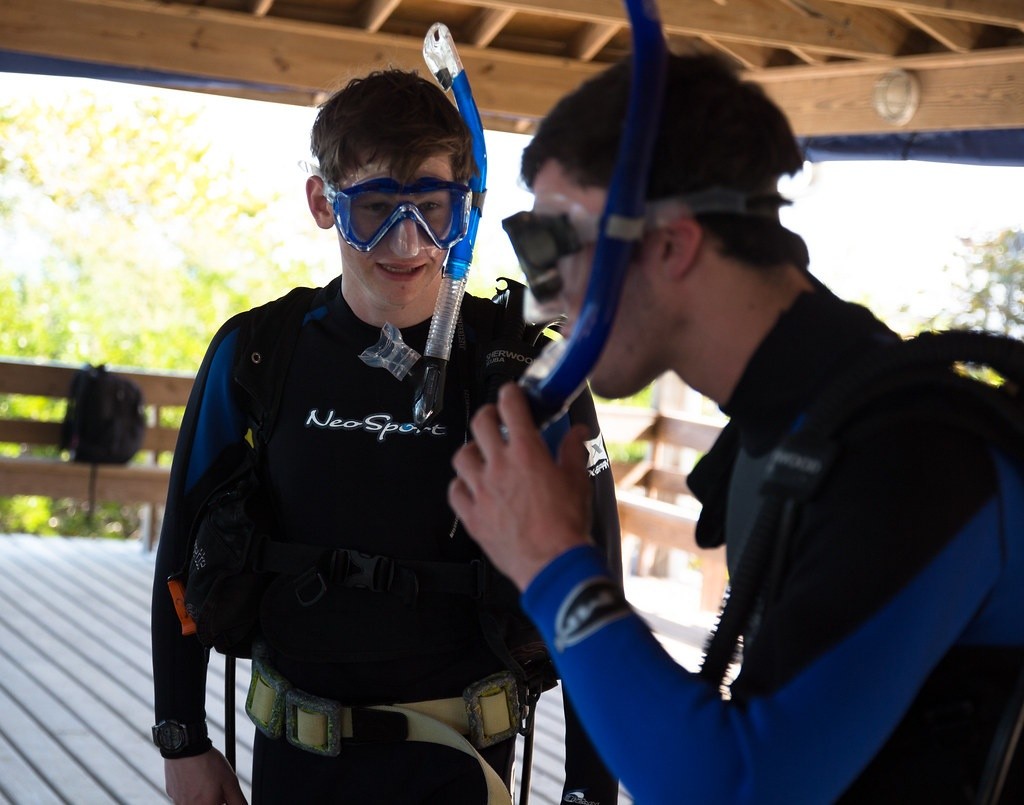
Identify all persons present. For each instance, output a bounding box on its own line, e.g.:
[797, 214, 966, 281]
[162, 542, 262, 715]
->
[447, 53, 1024, 804]
[151, 69, 623, 805]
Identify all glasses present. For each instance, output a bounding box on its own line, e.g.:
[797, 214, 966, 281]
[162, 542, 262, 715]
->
[324, 173, 474, 252]
[500, 194, 603, 306]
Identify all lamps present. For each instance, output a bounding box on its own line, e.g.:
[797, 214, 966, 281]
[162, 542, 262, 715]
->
[872, 72, 920, 126]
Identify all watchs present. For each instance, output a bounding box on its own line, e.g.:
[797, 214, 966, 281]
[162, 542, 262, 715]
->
[152, 721, 208, 754]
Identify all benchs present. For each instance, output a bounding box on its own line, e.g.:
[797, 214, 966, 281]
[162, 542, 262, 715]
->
[2, 359, 733, 613]
